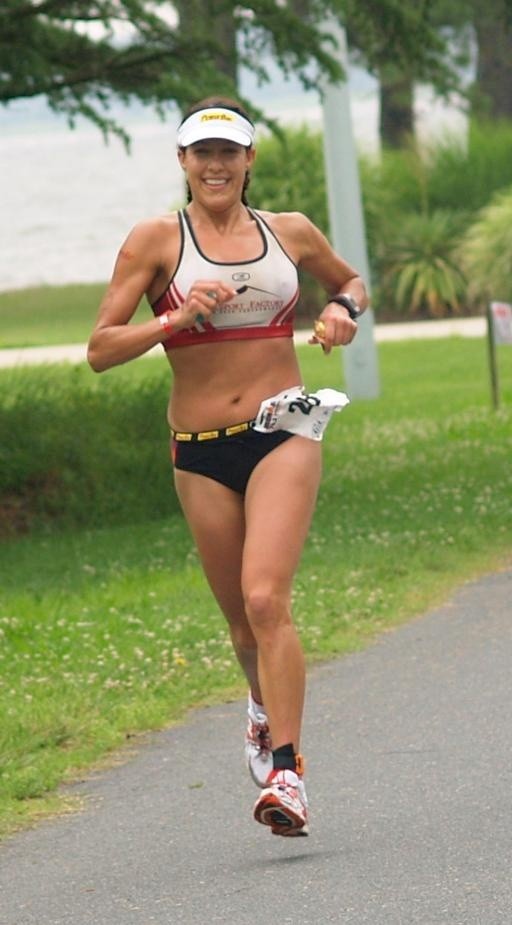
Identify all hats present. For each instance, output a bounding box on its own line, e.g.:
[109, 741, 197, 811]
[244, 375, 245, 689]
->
[177, 106, 255, 149]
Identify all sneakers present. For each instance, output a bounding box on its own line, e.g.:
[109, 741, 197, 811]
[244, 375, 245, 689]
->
[245, 690, 309, 837]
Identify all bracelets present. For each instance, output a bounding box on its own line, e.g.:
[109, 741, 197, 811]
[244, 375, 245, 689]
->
[161, 310, 179, 339]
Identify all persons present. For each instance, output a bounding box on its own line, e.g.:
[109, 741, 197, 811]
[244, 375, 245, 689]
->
[87, 96, 369, 837]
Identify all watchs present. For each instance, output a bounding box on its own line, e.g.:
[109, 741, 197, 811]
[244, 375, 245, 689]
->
[329, 293, 360, 319]
